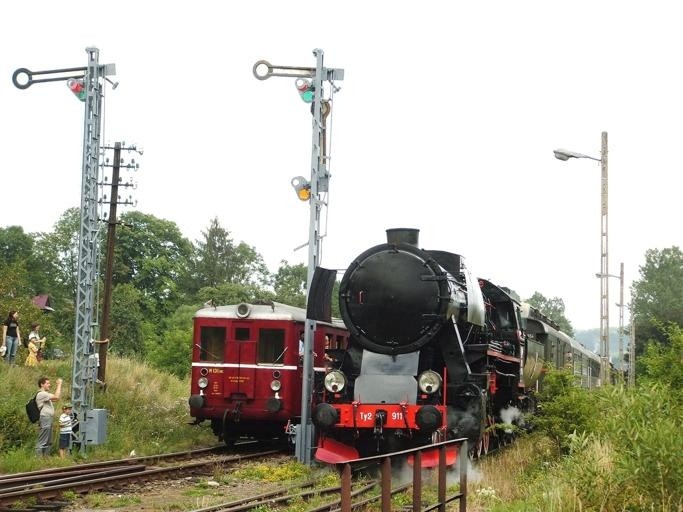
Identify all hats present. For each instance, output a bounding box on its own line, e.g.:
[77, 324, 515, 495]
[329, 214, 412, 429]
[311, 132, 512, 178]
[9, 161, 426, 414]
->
[62, 403, 74, 410]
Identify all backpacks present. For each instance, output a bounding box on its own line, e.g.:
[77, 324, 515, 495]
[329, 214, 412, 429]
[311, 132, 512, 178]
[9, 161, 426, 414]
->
[26, 391, 43, 424]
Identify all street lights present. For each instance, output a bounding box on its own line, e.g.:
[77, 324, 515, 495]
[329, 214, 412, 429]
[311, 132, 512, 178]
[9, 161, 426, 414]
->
[553, 130, 636, 389]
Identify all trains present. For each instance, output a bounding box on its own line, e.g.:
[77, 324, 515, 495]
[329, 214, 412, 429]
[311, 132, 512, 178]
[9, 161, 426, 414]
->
[311, 228, 618, 478]
[187, 297, 364, 450]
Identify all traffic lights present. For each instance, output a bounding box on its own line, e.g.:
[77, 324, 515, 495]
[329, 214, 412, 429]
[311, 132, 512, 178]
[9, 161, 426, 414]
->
[69, 80, 88, 101]
[292, 178, 310, 202]
[296, 79, 314, 103]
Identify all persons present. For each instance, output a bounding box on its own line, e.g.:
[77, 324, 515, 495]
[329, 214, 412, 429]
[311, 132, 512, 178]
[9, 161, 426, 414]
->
[324, 335, 334, 362]
[25, 334, 38, 368]
[2, 311, 21, 366]
[298, 332, 318, 361]
[58, 403, 74, 458]
[34, 377, 63, 458]
[29, 322, 46, 362]
[83, 330, 108, 384]
